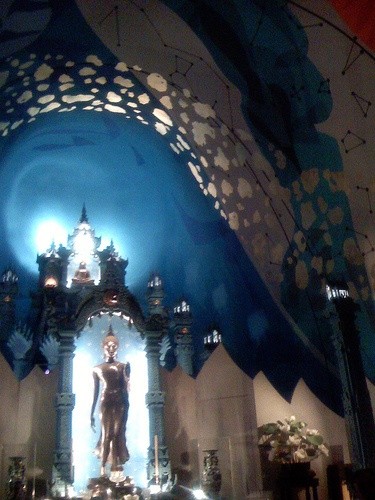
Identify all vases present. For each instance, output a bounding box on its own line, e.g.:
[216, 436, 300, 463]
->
[201, 448, 224, 500]
[7, 456, 30, 500]
[264, 459, 312, 491]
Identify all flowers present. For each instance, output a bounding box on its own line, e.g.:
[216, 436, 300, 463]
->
[256, 415, 331, 465]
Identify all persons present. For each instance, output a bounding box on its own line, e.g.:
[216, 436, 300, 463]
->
[72, 262, 95, 284]
[91, 484, 144, 500]
[89, 324, 131, 478]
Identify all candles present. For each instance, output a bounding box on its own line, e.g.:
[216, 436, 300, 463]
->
[152, 434, 163, 485]
